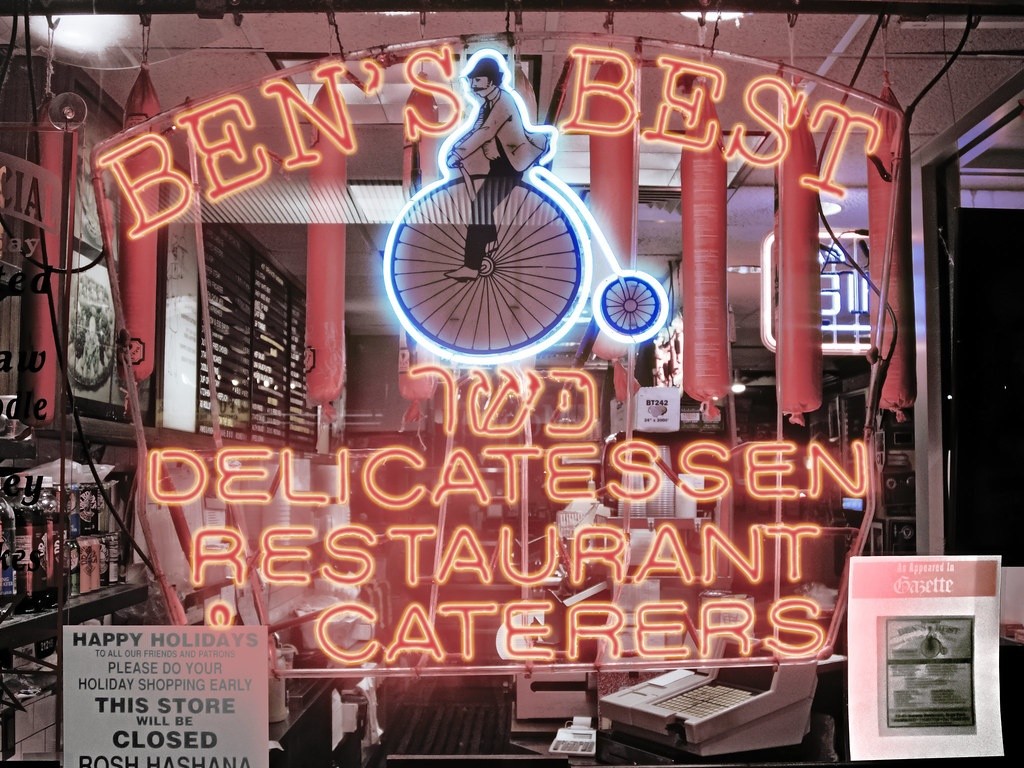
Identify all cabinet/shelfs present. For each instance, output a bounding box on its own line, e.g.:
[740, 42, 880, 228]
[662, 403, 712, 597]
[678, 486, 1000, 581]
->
[1, 436, 150, 768]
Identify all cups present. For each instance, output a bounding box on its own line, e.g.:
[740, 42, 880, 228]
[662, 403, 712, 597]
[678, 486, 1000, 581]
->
[230, 460, 349, 547]
[279, 649, 296, 679]
[620, 445, 706, 518]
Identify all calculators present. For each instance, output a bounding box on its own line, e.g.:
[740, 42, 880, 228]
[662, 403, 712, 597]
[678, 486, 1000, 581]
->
[547, 728, 596, 756]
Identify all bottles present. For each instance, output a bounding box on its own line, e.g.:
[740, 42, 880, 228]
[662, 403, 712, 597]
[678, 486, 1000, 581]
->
[0, 477, 59, 597]
[268, 650, 286, 721]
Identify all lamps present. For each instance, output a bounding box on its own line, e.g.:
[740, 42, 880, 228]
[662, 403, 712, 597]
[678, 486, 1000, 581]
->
[42, 92, 88, 130]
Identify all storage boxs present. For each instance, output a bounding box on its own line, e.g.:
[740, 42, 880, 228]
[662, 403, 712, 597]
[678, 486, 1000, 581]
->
[610, 386, 680, 433]
